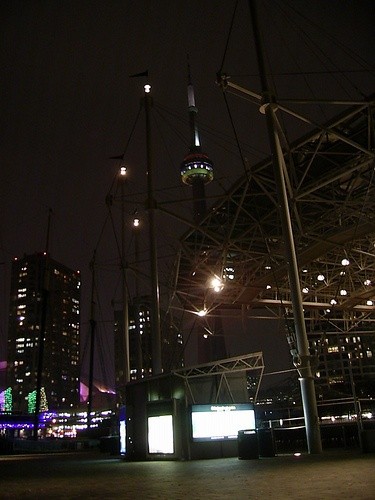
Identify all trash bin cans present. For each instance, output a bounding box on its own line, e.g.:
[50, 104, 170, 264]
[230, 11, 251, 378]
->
[237, 429, 259, 460]
[256, 428, 275, 456]
